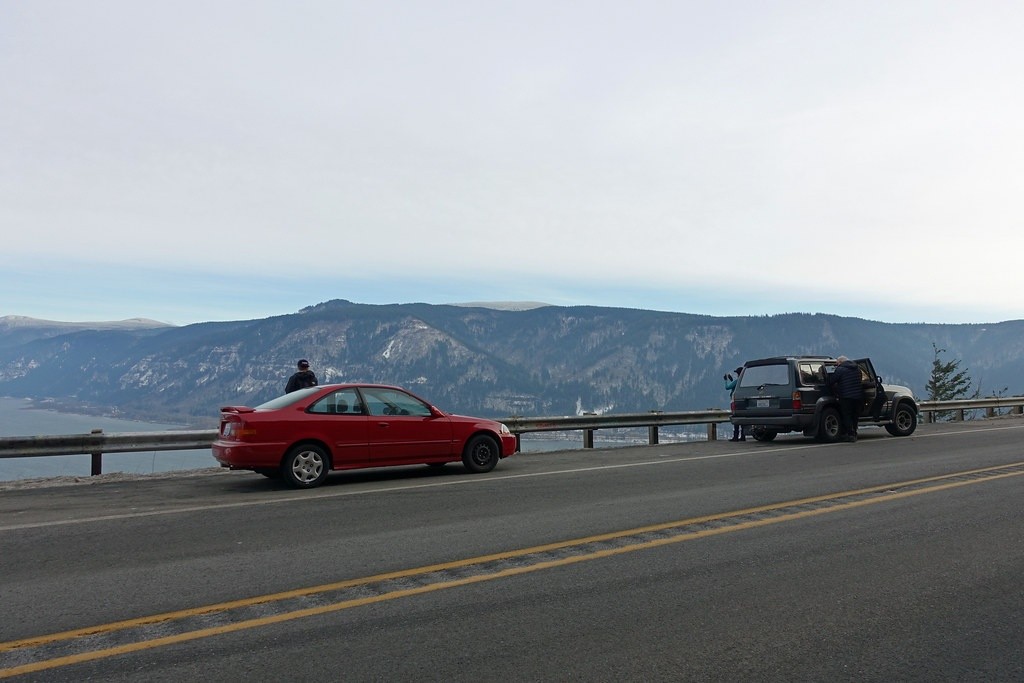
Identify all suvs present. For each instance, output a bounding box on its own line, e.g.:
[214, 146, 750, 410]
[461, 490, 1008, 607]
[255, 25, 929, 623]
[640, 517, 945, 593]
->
[730, 356, 920, 443]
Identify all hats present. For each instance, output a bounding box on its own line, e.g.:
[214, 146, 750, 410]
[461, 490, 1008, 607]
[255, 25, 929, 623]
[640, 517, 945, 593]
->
[297, 360, 309, 367]
[734, 367, 743, 374]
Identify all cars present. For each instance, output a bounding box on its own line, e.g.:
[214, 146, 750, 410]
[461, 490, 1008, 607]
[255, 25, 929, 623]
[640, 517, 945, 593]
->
[212, 383, 517, 489]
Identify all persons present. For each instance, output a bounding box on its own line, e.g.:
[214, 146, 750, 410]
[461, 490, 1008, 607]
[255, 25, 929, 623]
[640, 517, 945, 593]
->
[285, 360, 318, 394]
[830, 356, 862, 442]
[723, 367, 746, 442]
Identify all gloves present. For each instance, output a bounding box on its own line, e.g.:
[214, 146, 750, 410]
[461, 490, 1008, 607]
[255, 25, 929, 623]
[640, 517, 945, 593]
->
[728, 374, 733, 381]
[723, 373, 727, 380]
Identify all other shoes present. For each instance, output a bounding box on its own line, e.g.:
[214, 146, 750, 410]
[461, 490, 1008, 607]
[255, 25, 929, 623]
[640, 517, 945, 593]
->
[840, 433, 858, 442]
[729, 431, 739, 442]
[739, 433, 746, 441]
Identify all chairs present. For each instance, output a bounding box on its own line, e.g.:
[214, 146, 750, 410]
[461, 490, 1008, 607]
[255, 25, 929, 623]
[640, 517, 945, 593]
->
[337, 400, 348, 413]
[353, 398, 361, 413]
